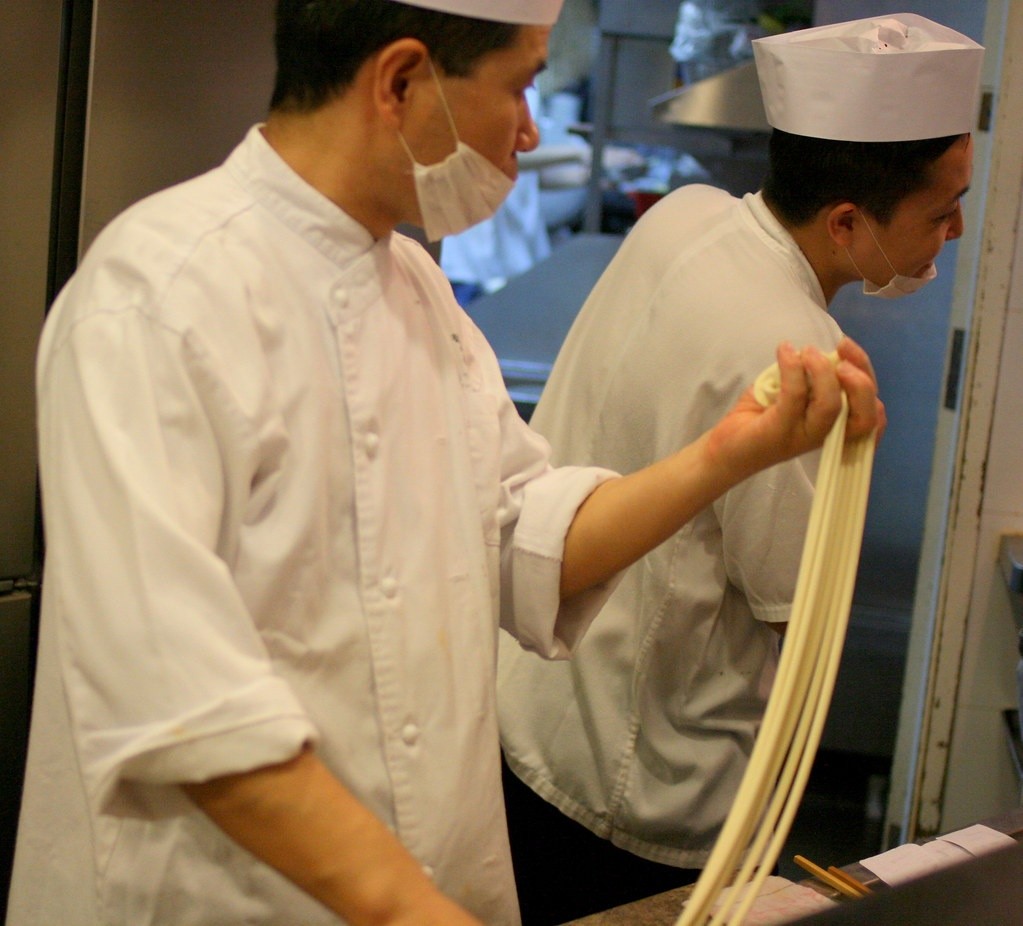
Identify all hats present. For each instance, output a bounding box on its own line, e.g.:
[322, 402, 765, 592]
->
[751, 13, 986, 142]
[396, 0, 564, 25]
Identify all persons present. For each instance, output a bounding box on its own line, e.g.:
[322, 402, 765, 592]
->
[0, 0, 889, 926]
[490, 10, 983, 926]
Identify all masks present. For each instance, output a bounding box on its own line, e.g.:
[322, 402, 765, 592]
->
[395, 62, 515, 243]
[844, 207, 937, 298]
